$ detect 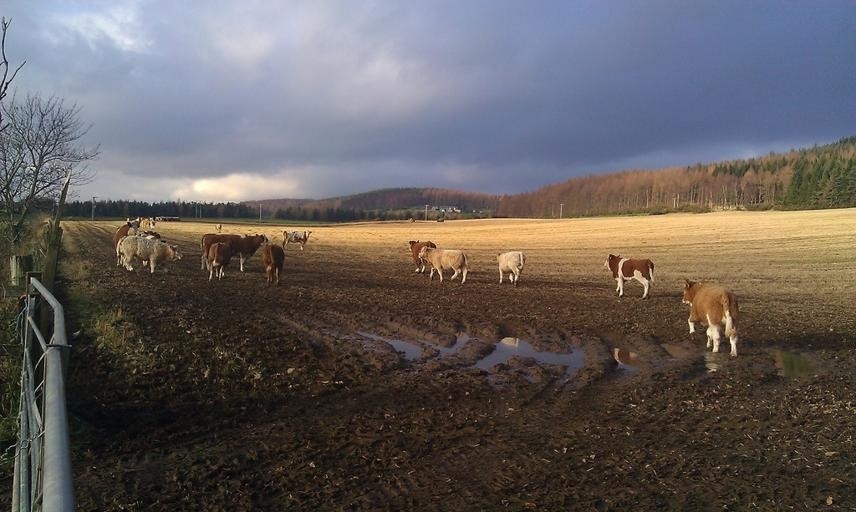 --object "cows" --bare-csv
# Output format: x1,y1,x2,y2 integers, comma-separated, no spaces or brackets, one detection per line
112,217,184,275
214,224,222,233
497,251,526,288
282,229,312,251
409,239,436,274
200,232,269,282
263,243,285,288
603,253,656,300
418,245,470,285
682,275,739,361
